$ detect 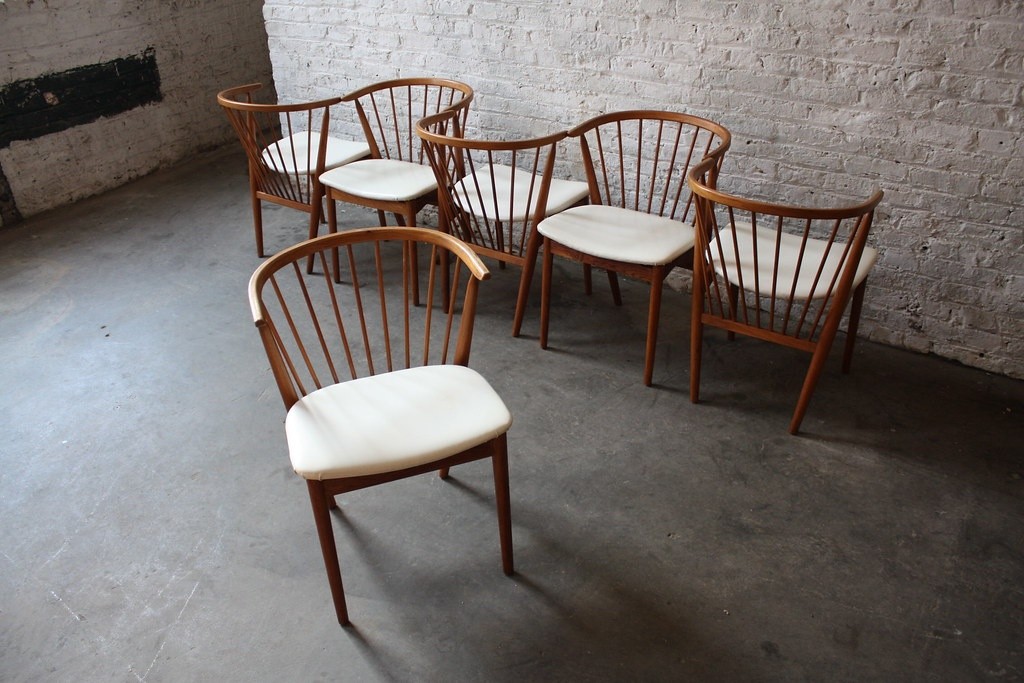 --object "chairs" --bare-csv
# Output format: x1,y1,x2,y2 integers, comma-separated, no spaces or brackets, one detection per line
685,156,885,435
415,109,593,338
215,83,390,275
318,77,475,308
536,110,730,387
248,227,513,626
